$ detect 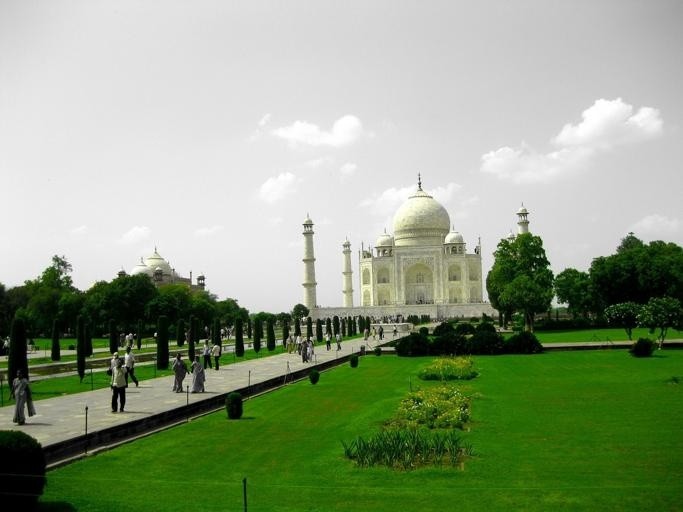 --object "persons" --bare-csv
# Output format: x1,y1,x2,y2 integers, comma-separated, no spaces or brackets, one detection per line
108,332,139,412
172,327,232,394
8,370,29,425
362,325,397,342
2,335,11,356
286,331,341,364
0,429,78,511
